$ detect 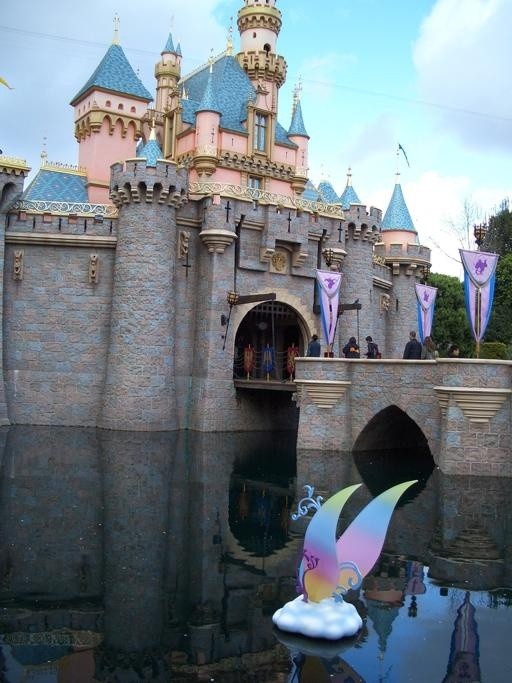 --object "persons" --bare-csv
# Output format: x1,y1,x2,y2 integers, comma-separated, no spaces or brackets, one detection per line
403,331,422,359
362,336,382,359
305,334,321,357
421,336,437,360
342,336,361,359
447,345,462,358
